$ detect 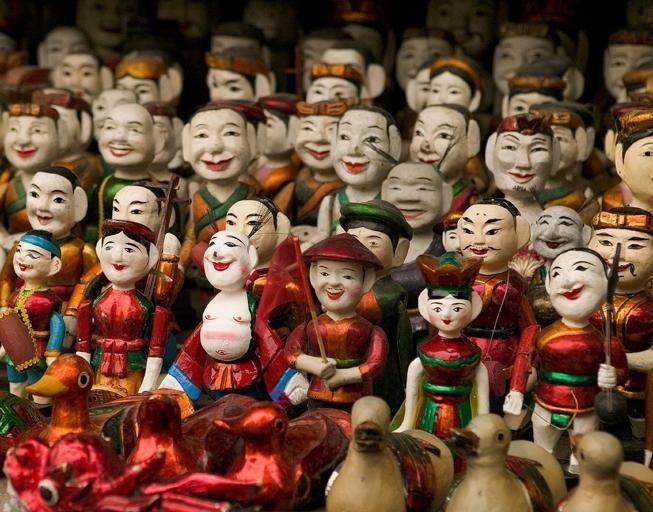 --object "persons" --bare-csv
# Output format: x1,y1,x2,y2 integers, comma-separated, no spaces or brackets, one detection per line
1,1,653,486
0,1,653,512
